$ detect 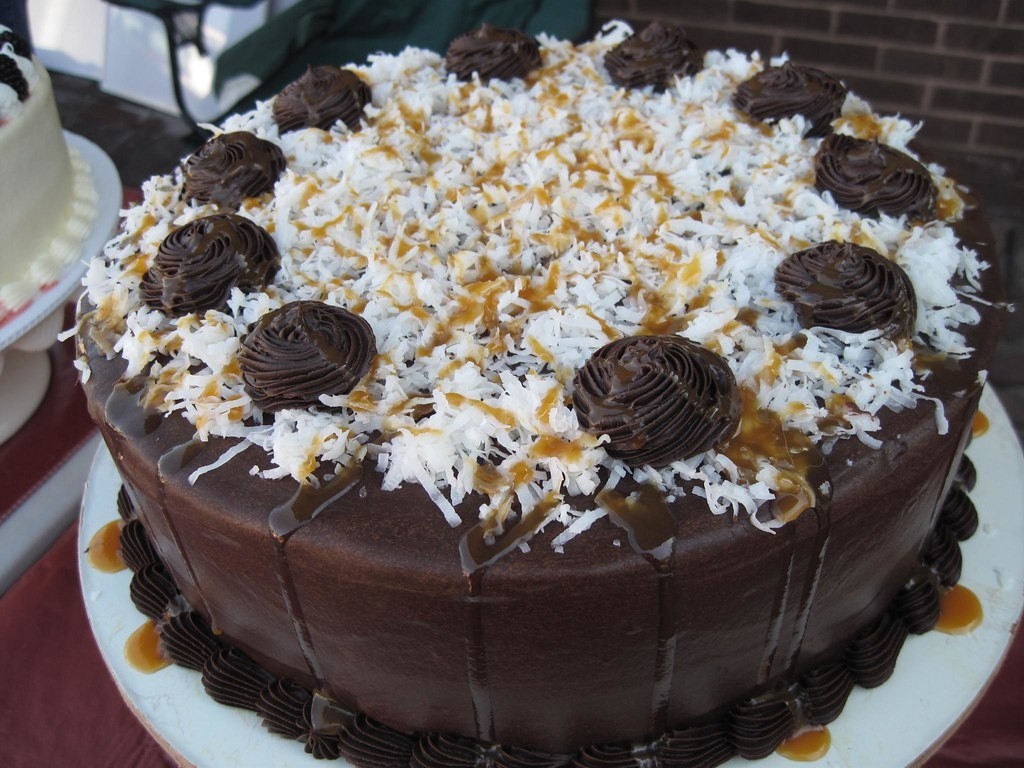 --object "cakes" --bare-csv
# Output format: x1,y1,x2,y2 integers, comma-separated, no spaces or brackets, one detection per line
52,15,1016,768
